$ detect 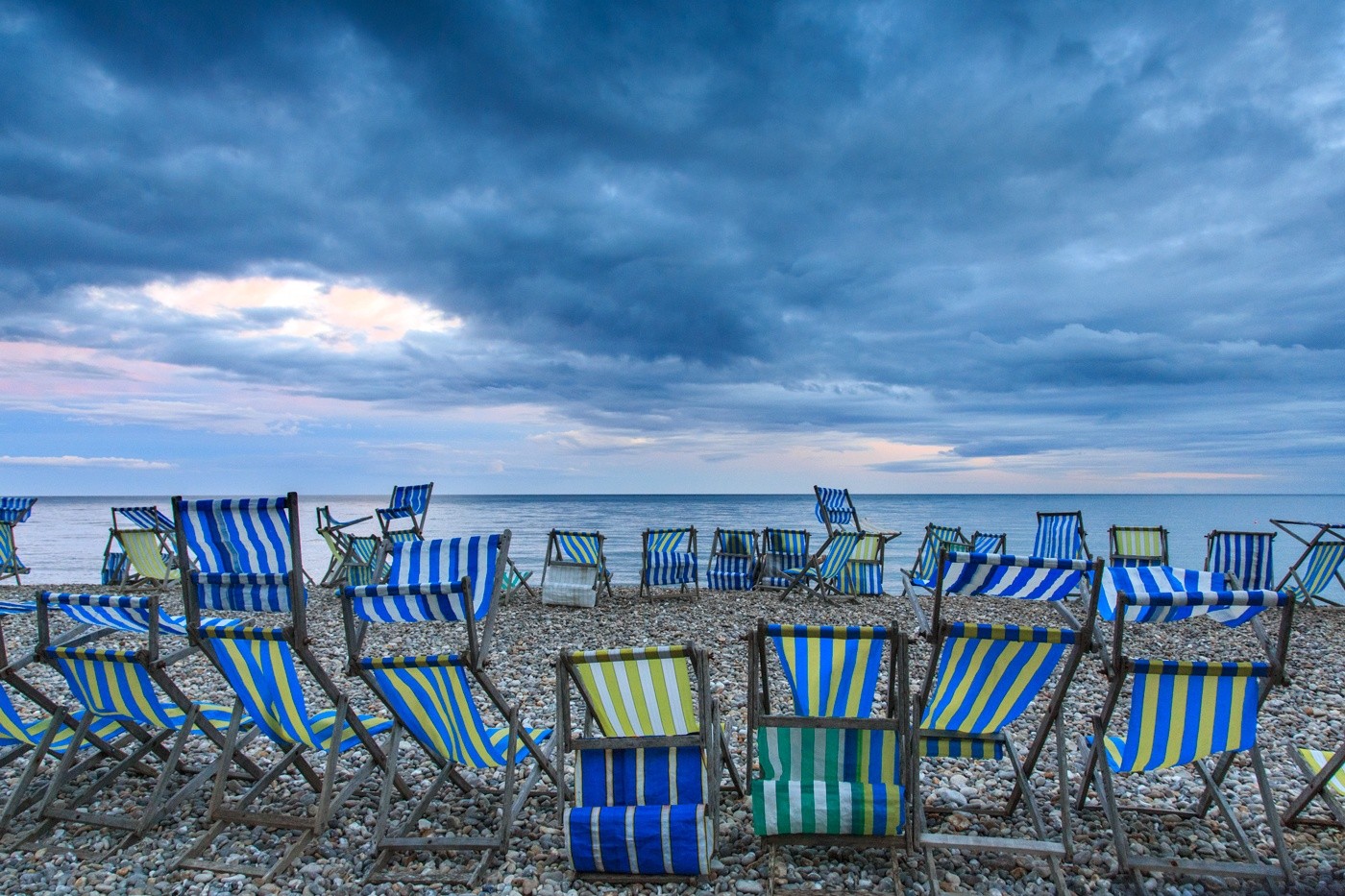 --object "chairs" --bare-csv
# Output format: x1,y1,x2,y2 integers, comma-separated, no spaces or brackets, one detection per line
0,482,1345,896
